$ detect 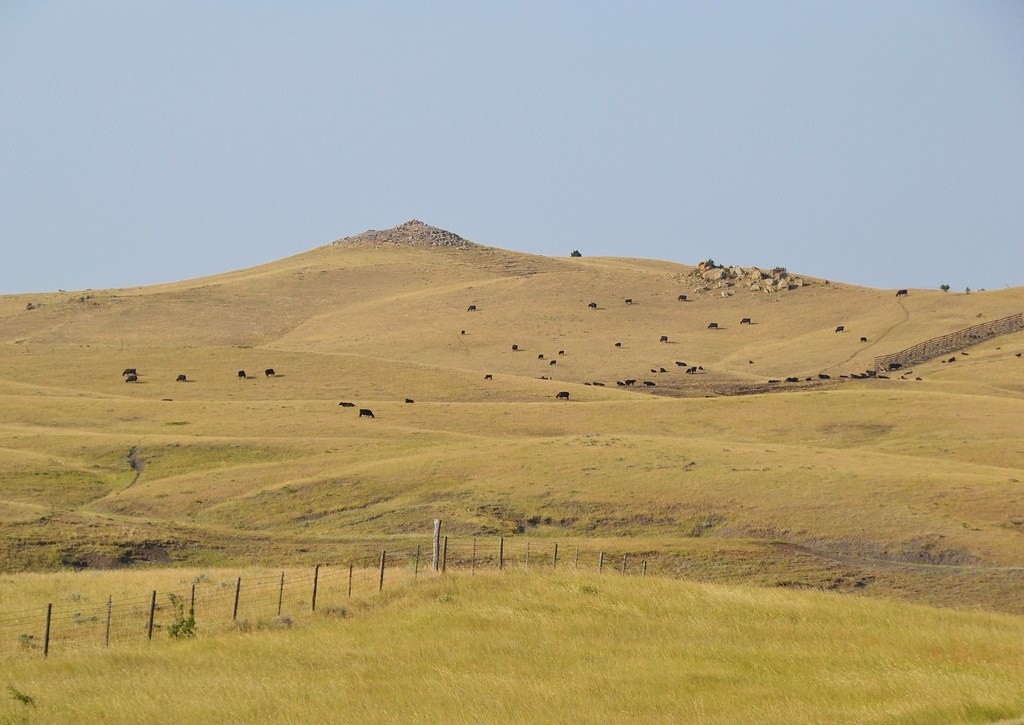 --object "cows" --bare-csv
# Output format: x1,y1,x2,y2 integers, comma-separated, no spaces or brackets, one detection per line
123,289,1021,418
